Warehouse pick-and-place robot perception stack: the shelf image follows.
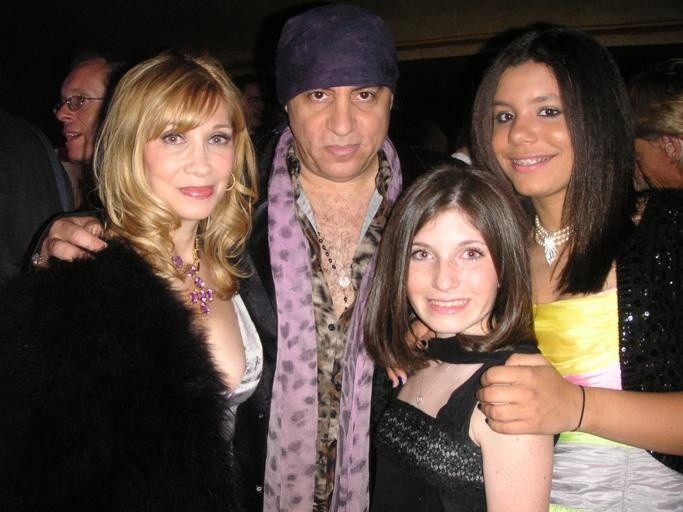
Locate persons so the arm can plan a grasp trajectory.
[1,61,275,284]
[1,50,280,512]
[32,4,474,511]
[471,21,683,511]
[364,165,554,511]
[388,59,682,187]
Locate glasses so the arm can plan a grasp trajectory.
[53,95,111,115]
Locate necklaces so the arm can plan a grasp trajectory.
[170,236,214,321]
